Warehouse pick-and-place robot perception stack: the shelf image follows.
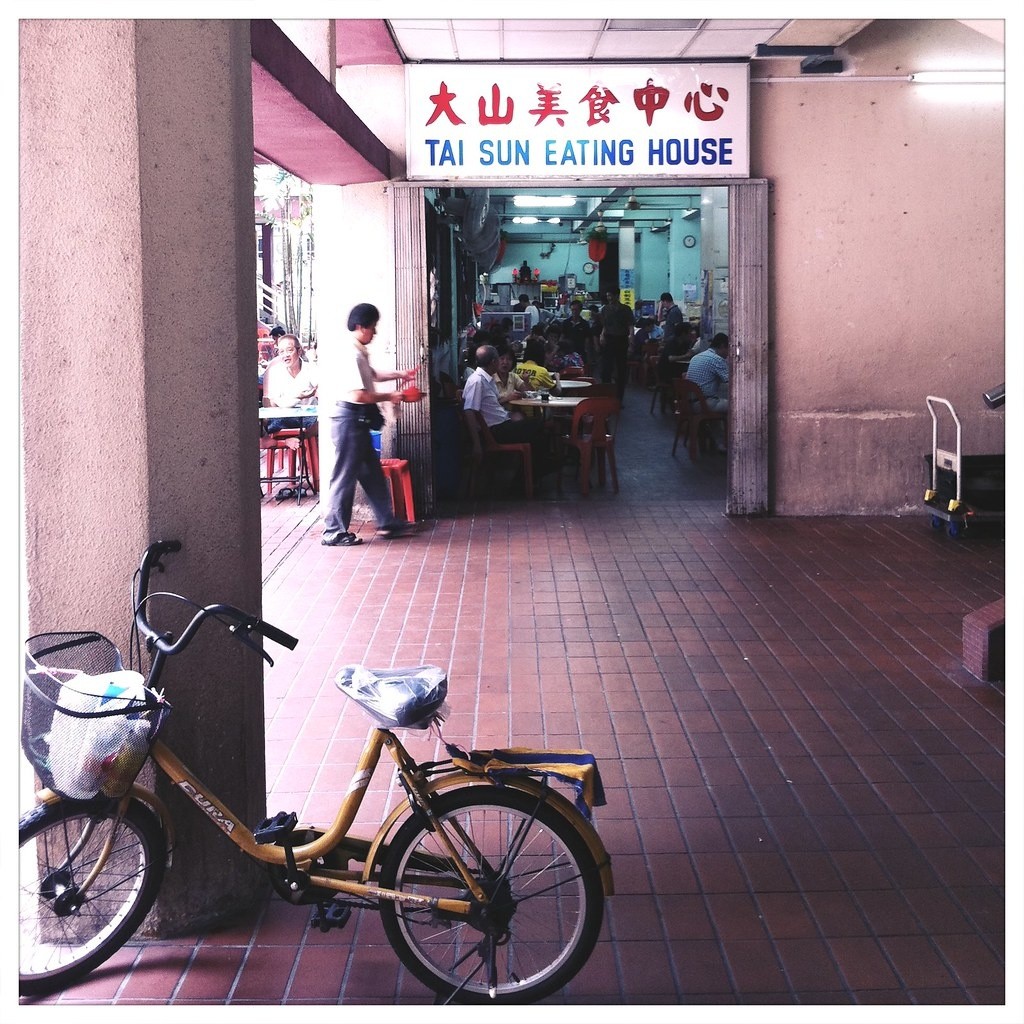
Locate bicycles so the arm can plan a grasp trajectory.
[17,541,616,1005]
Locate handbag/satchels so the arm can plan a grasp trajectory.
[356,404,385,431]
[48,670,146,799]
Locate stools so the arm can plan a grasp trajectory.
[266,427,321,494]
[374,458,416,524]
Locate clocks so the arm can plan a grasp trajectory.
[683,235,696,247]
[584,263,595,274]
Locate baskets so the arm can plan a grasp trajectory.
[20,630,172,804]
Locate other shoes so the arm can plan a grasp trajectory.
[620,404,625,409]
[321,532,363,546]
[376,521,416,539]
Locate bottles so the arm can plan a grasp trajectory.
[101,718,152,798]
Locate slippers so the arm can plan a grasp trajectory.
[293,486,306,498]
[275,487,293,499]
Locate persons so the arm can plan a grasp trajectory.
[685,332,728,453]
[259,326,286,384]
[263,335,316,450]
[321,304,419,546]
[462,287,700,477]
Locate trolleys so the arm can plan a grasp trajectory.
[923,396,1005,537]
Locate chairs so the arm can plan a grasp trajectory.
[458,341,729,499]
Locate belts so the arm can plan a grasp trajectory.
[337,400,362,411]
[693,396,712,403]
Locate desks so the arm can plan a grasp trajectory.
[549,379,593,397]
[507,394,589,473]
[257,405,317,505]
